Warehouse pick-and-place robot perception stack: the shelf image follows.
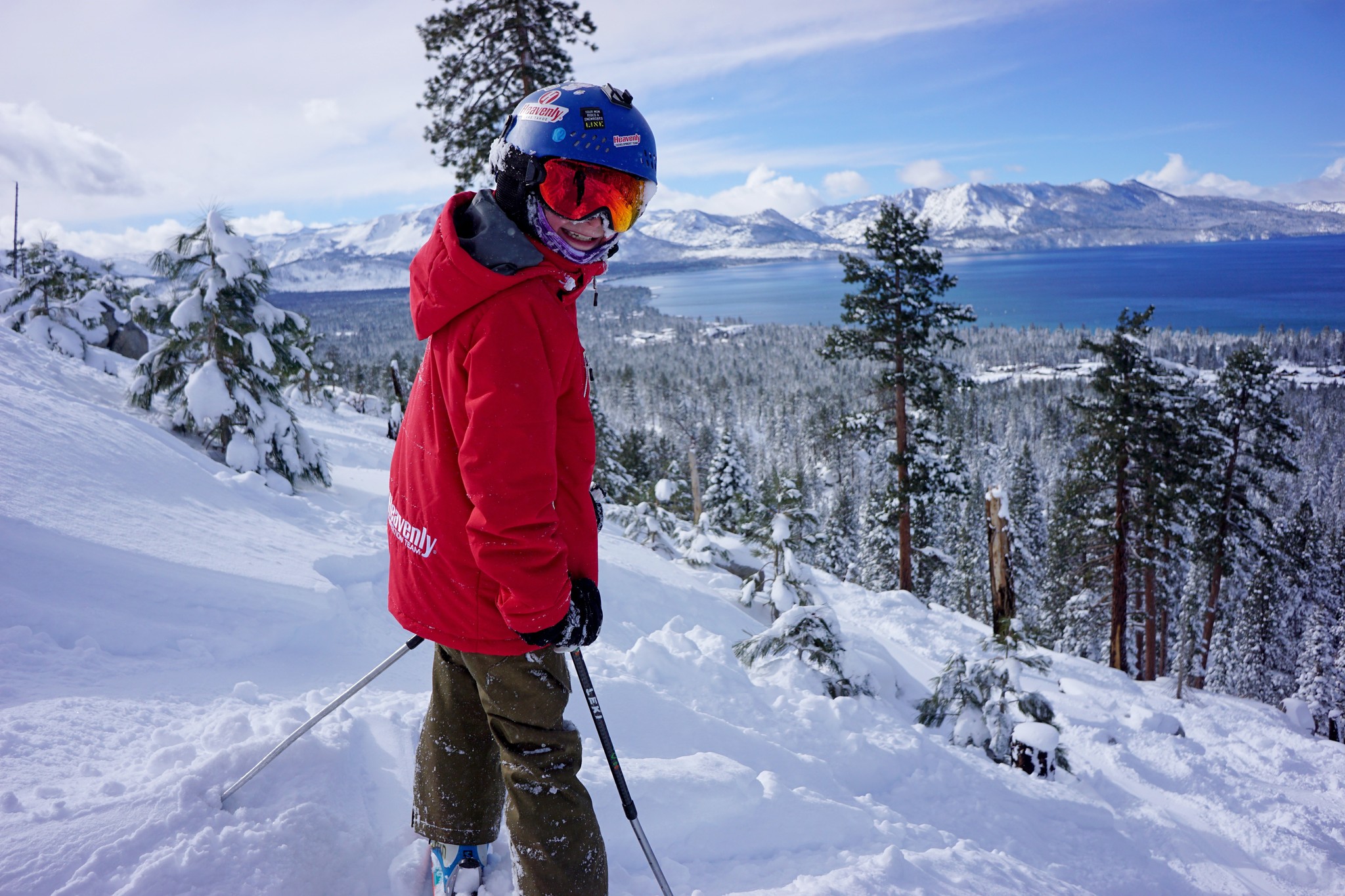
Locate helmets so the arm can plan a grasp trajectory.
[485,80,659,263]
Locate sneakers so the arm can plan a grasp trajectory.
[423,831,496,896]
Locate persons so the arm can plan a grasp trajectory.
[385,78,661,896]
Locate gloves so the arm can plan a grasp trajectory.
[527,573,605,653]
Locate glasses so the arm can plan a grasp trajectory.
[536,159,653,232]
